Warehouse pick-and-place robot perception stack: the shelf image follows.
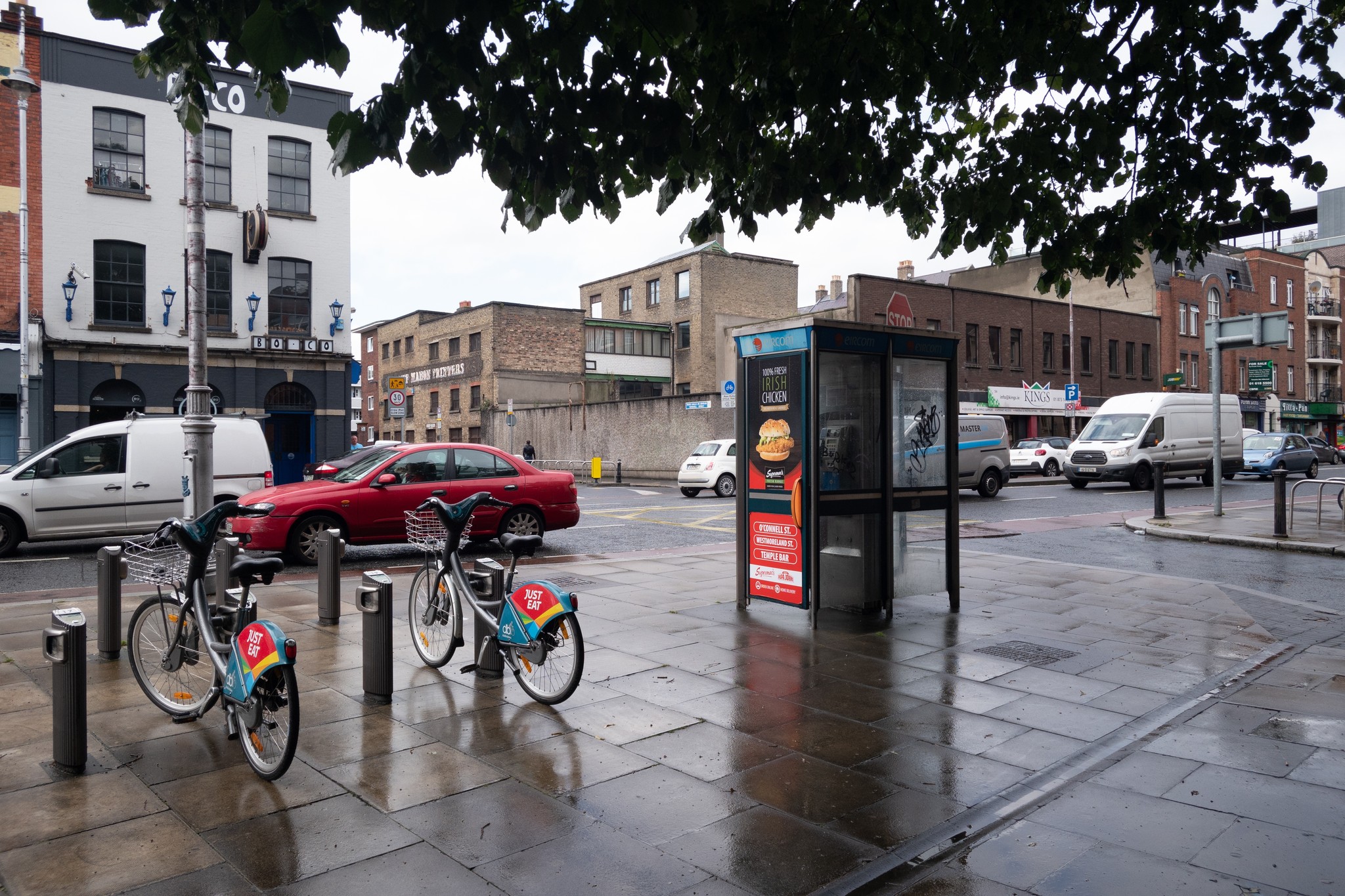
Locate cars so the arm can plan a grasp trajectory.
[1008,436,1073,480]
[1338,441,1345,464]
[224,440,580,569]
[1304,435,1340,465]
[302,439,477,482]
[1242,428,1319,481]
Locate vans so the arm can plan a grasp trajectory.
[1060,392,1243,493]
[677,439,736,498]
[891,413,1012,500]
[0,406,281,561]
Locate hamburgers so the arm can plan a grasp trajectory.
[756,419,794,461]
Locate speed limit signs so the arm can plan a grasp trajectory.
[389,390,405,406]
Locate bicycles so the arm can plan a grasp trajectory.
[403,490,585,706]
[122,496,302,781]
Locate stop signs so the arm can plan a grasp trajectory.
[886,291,913,327]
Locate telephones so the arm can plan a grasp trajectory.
[820,417,862,490]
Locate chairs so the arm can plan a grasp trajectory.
[421,459,437,482]
[1329,348,1338,359]
[1308,303,1316,316]
[1319,389,1333,402]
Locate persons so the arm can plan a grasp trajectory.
[351,436,363,450]
[388,456,426,484]
[1316,431,1327,447]
[83,440,120,474]
[523,440,536,464]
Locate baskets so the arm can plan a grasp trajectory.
[123,529,190,586]
[402,507,476,553]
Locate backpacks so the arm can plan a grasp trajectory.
[525,445,534,455]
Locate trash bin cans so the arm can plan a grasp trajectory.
[591,457,602,479]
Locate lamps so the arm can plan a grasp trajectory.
[161,284,177,327]
[61,279,78,322]
[328,298,344,336]
[245,291,262,332]
